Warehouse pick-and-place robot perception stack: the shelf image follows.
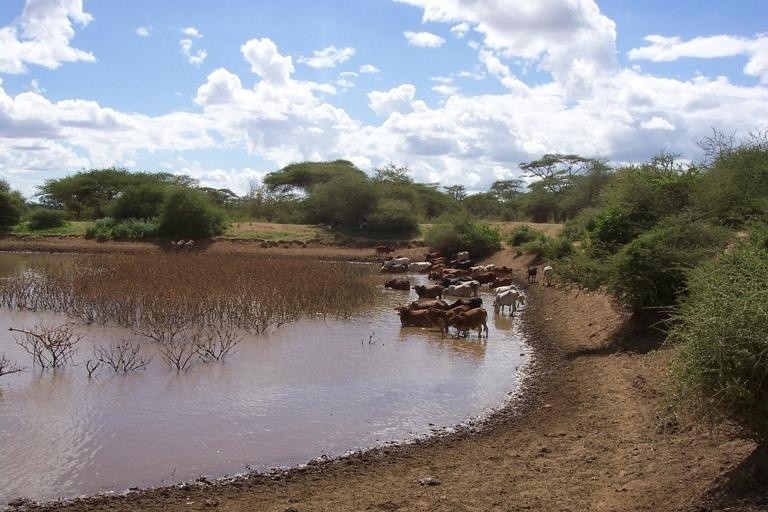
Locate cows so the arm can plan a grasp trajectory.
[543,266,553,287]
[379,251,526,338]
[183,240,195,249]
[375,246,395,257]
[526,267,537,284]
[171,241,177,249]
[177,239,185,248]
